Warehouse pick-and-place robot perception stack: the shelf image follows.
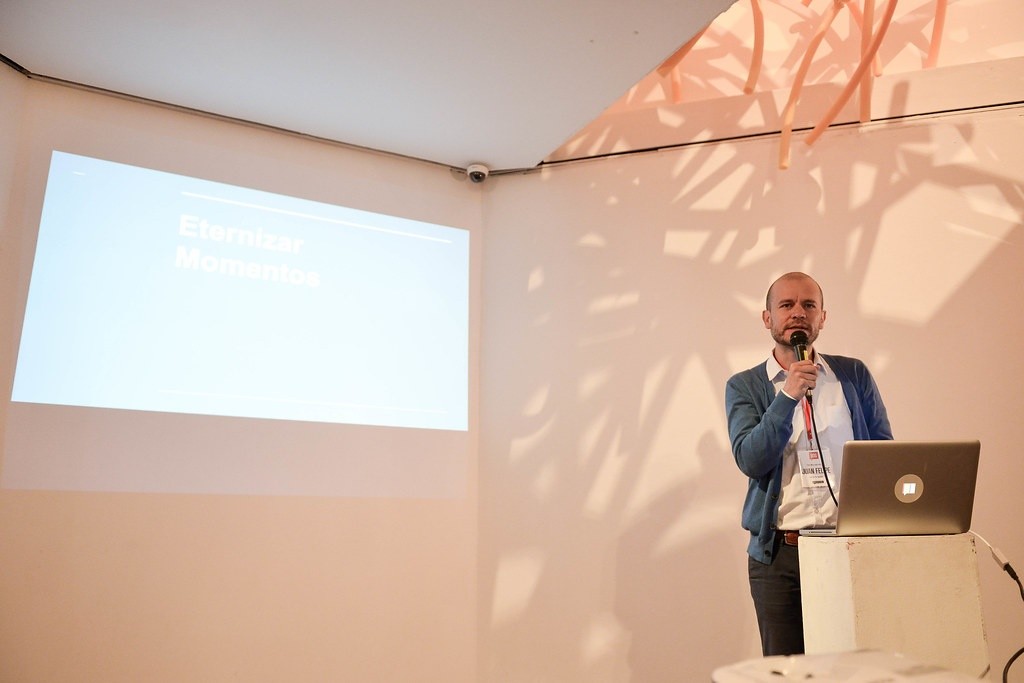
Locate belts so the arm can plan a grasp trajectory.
[773,530,801,546]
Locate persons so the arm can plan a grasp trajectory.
[724,272,894,658]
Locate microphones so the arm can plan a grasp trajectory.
[791,332,811,404]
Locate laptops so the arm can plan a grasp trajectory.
[799,439,982,536]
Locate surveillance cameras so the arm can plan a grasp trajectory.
[467,164,489,182]
